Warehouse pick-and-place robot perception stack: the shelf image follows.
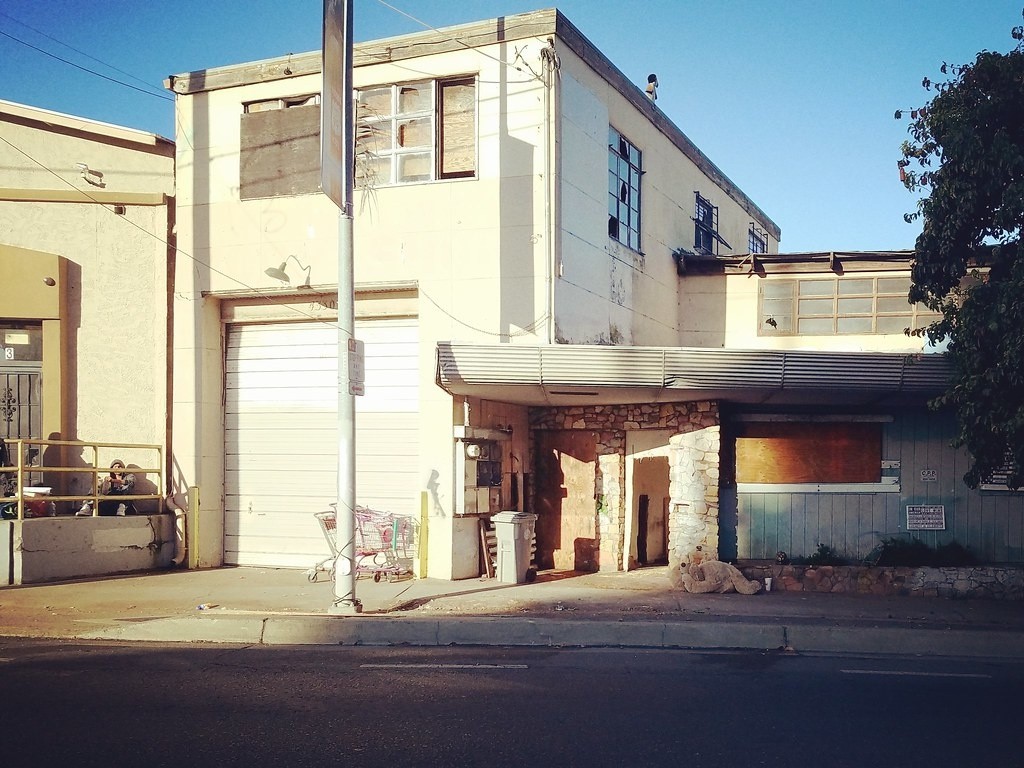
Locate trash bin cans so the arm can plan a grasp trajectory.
[489,511,538,585]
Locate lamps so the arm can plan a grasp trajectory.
[264,255,312,288]
[77,162,106,185]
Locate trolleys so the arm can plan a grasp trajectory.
[306,501,411,583]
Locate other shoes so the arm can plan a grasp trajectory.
[116,503,128,516]
[75,504,92,516]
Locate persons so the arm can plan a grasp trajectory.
[645,74,658,104]
[76,459,136,517]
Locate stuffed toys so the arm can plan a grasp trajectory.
[671,546,762,595]
